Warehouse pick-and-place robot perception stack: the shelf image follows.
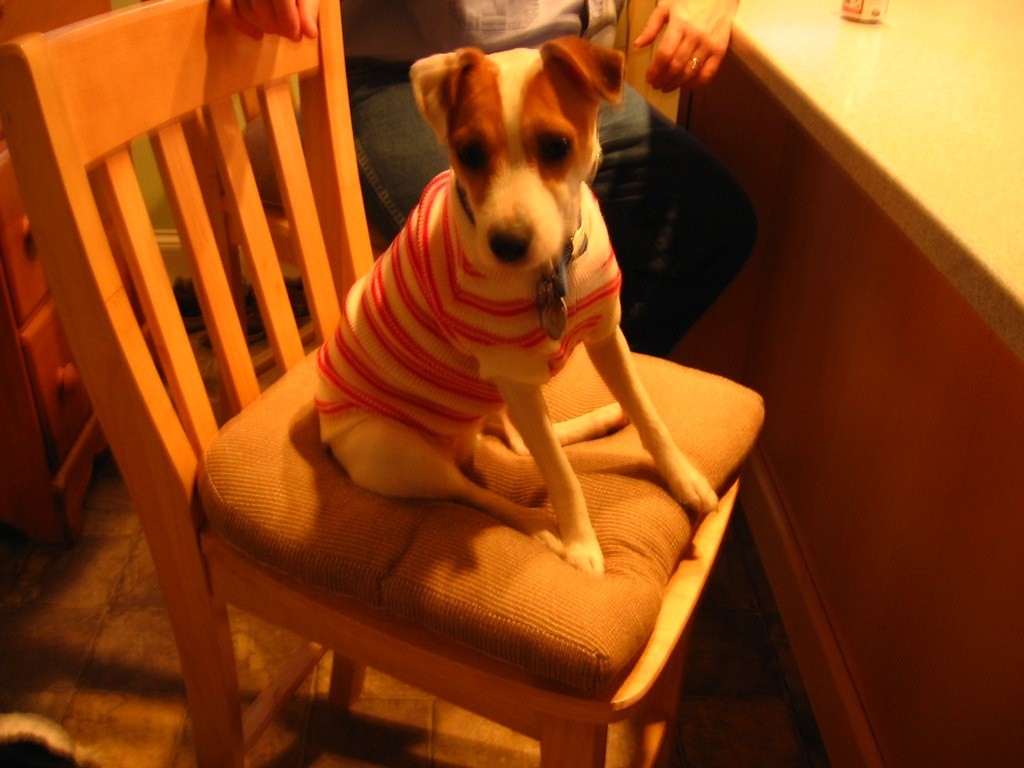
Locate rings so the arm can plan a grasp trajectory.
[690,57,703,70]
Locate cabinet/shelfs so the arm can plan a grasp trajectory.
[0,137,149,544]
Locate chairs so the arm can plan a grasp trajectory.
[0,0,766,768]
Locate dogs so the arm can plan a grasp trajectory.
[313,35,722,576]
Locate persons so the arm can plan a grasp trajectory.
[214,0,760,367]
